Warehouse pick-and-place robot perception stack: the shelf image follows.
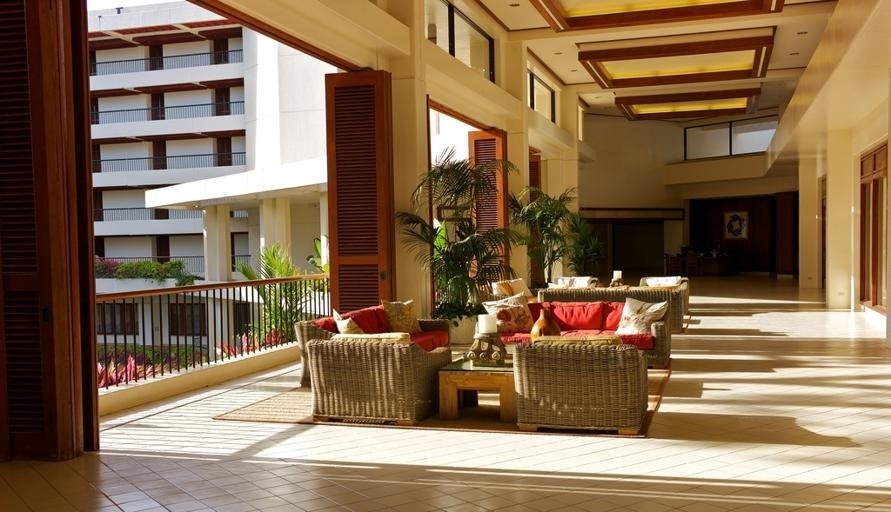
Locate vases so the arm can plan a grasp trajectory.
[530,308,560,342]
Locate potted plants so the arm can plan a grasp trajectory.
[387,146,532,343]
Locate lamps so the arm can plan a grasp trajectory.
[608,270,623,287]
[466,314,507,367]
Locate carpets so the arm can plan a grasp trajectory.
[210,357,672,440]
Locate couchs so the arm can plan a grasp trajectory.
[539,288,684,333]
[500,301,672,368]
[295,303,451,388]
[480,278,536,300]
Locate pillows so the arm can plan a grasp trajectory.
[490,281,512,296]
[332,309,366,334]
[506,279,532,297]
[614,297,669,335]
[479,292,534,332]
[382,299,424,333]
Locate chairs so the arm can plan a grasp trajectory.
[548,277,607,288]
[663,253,699,276]
[518,339,649,435]
[304,339,453,423]
[640,276,690,314]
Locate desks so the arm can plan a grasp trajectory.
[438,352,517,419]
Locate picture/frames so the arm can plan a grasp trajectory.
[723,211,749,239]
